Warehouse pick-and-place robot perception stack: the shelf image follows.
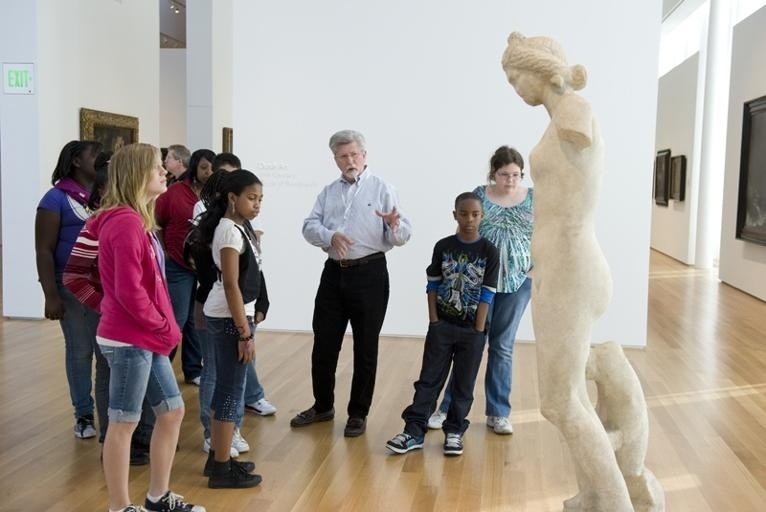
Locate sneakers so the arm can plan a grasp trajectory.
[143,494,207,512]
[427,409,446,428]
[108,503,146,512]
[245,399,276,416]
[183,371,204,385]
[487,414,515,436]
[130,448,147,466]
[386,434,426,451]
[201,436,239,456]
[209,469,260,491]
[229,432,252,449]
[201,459,256,474]
[132,434,178,451]
[75,419,95,439]
[442,428,462,456]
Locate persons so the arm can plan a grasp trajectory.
[385,193,499,456]
[84,144,207,512]
[204,169,263,488]
[154,149,216,386]
[62,150,180,466]
[426,147,533,435]
[166,144,191,180]
[290,131,412,437]
[501,31,666,512]
[183,169,270,458]
[36,141,104,439]
[193,153,278,416]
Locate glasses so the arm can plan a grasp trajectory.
[335,151,365,160]
[496,172,520,178]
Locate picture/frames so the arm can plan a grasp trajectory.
[80,109,139,168]
[656,150,668,206]
[735,94,766,243]
[669,155,687,202]
[223,127,232,156]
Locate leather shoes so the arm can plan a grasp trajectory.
[343,415,368,436]
[291,408,336,427]
